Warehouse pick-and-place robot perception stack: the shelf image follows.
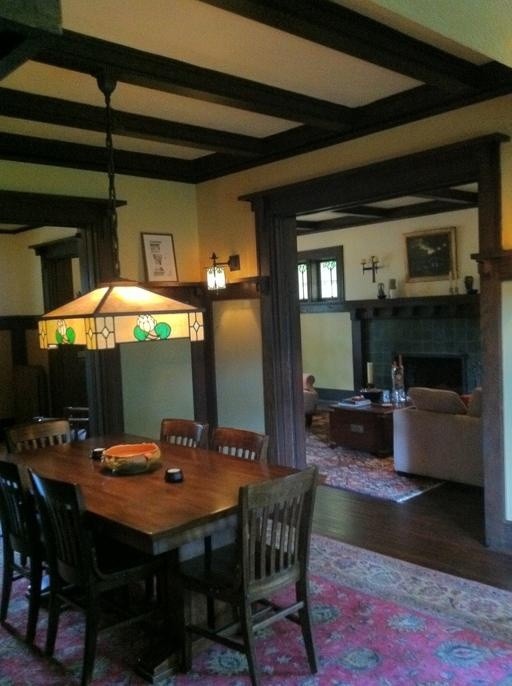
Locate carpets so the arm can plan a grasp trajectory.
[302,411,447,505]
[1,519,511,686]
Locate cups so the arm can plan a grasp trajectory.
[92,448,105,459]
[164,468,183,483]
[383,390,409,403]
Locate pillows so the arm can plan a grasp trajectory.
[467,387,483,418]
[406,387,468,415]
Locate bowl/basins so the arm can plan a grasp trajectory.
[101,442,162,474]
[359,391,383,403]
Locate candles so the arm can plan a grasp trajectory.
[454,269,459,288]
[449,269,453,289]
[366,362,374,384]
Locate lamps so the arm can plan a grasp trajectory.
[360,254,382,282]
[205,252,242,293]
[37,63,209,352]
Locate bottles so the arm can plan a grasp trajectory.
[388,278,397,299]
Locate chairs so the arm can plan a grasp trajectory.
[302,372,319,427]
[164,466,318,686]
[160,418,270,462]
[1,418,171,686]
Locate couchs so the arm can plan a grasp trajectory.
[392,395,484,489]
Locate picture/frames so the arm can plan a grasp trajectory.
[399,226,460,283]
[141,231,180,283]
